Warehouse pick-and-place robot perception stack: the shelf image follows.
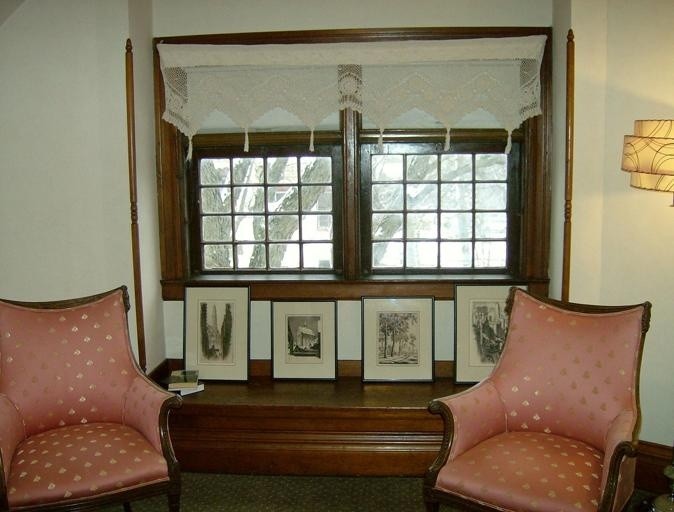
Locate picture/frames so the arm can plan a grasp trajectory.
[455,285,526,383]
[361,296,435,380]
[184,287,251,381]
[271,300,337,380]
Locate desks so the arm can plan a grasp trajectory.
[149,358,478,512]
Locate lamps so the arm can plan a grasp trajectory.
[622,120,674,191]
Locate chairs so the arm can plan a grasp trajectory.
[1,286,181,511]
[422,287,652,511]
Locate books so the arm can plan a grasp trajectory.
[168,370,205,397]
